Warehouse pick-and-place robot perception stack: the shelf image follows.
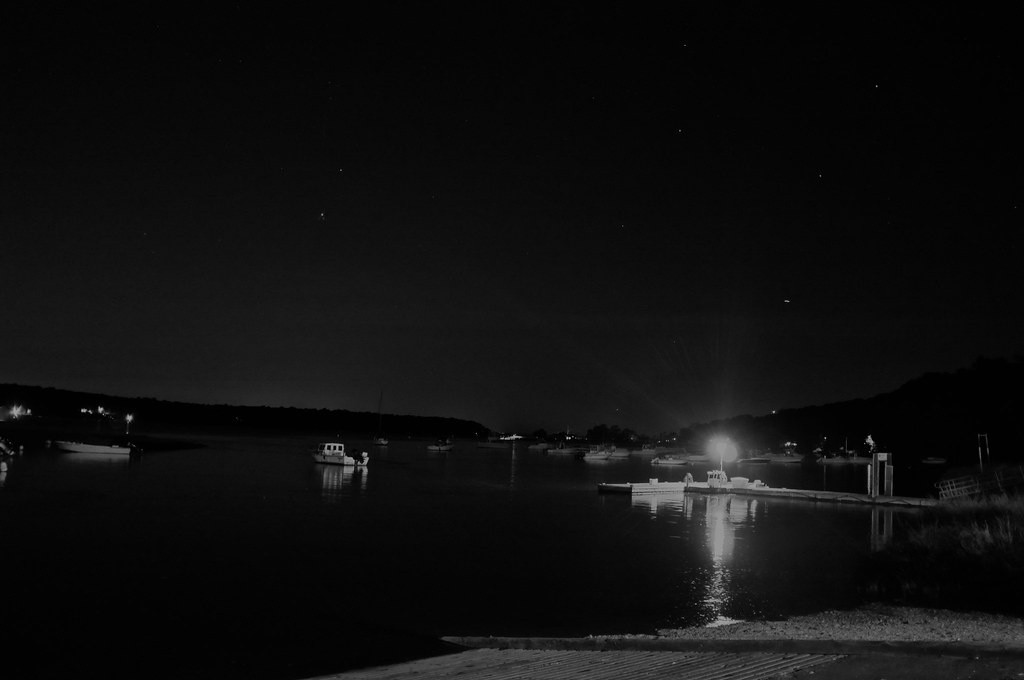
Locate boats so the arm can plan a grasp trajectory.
[584,452,612,461]
[673,453,773,469]
[803,437,872,465]
[651,456,687,469]
[313,442,370,468]
[528,442,553,451]
[371,435,389,446]
[56,439,147,457]
[427,438,455,452]
[476,436,510,449]
[610,449,631,457]
[544,443,590,460]
